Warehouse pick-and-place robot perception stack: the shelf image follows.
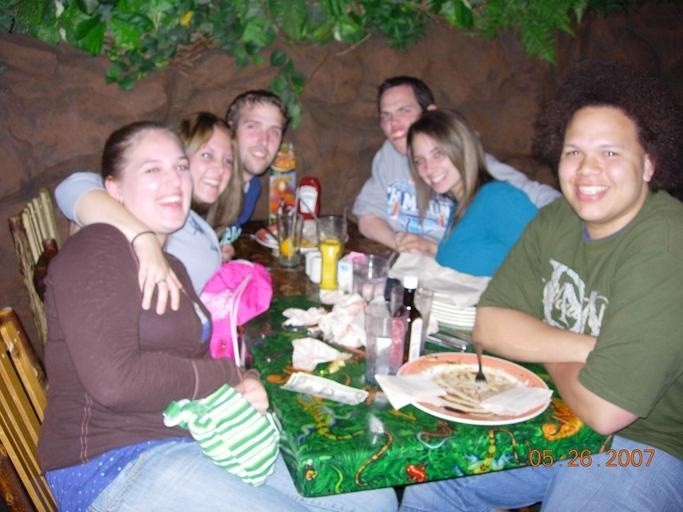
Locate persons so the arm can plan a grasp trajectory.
[390,107,539,309]
[213,88,294,263]
[30,118,399,512]
[50,107,243,316]
[398,59,683,512]
[348,72,566,259]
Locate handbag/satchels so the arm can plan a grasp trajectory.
[161,383,282,487]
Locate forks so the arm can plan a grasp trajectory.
[473,341,490,387]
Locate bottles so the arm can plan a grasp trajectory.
[391,275,423,369]
[268,140,322,221]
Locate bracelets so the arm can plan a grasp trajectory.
[128,231,157,248]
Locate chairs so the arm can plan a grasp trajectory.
[0,306,61,512]
[6,187,62,358]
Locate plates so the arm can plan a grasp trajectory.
[397,351,552,427]
[255,219,348,249]
[411,291,477,334]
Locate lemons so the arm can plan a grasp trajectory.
[280,237,294,257]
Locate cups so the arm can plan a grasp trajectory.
[275,215,412,384]
